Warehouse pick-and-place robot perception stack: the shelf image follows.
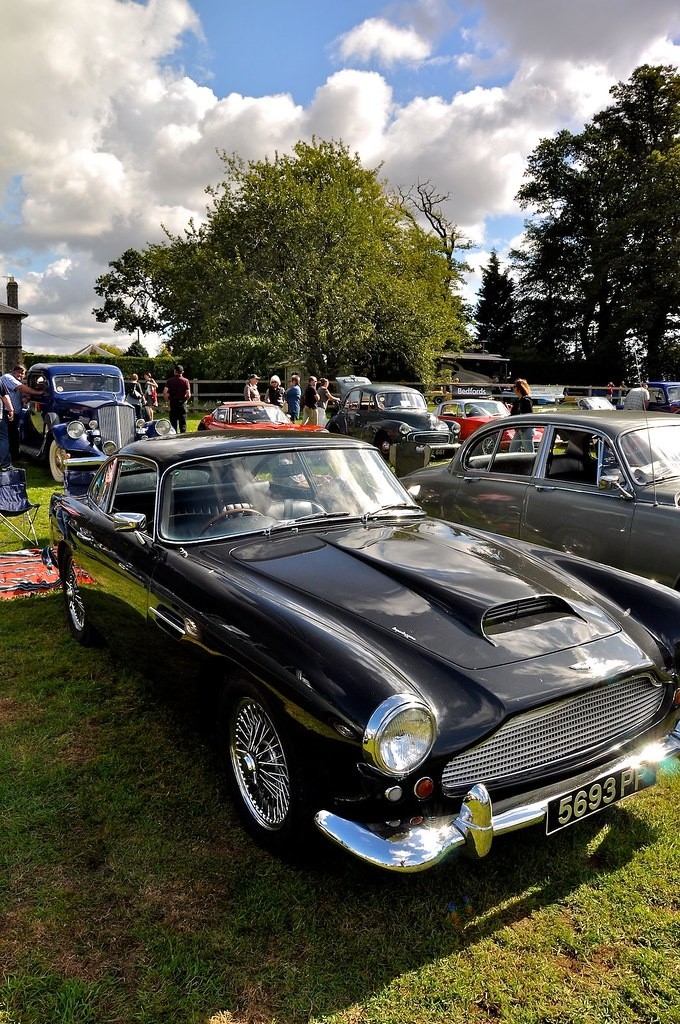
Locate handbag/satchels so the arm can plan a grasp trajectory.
[129,384,142,399]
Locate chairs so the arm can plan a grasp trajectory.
[469,407,486,417]
[382,394,402,409]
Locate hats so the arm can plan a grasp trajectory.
[248,374,260,379]
[270,375,281,386]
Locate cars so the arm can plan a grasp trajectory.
[198,401,329,432]
[18,363,176,484]
[432,398,563,453]
[325,375,462,462]
[398,409,680,592]
[47,429,680,874]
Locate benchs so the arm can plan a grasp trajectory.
[471,454,598,485]
[140,493,327,539]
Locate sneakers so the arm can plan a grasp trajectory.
[1,465,16,471]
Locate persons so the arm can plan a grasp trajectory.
[244,374,261,402]
[163,365,192,434]
[142,372,158,422]
[125,373,146,419]
[264,375,340,427]
[607,382,615,402]
[504,383,512,409]
[623,383,650,411]
[617,381,627,405]
[493,378,502,400]
[0,365,46,472]
[271,451,333,490]
[508,379,534,453]
